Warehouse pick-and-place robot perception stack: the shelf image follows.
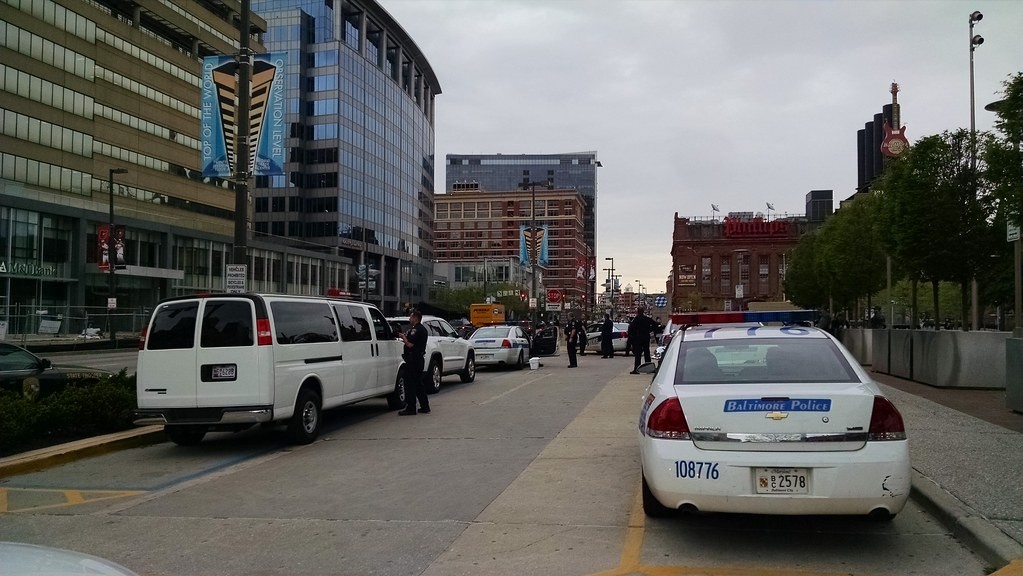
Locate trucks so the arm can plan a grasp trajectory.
[471,304,510,330]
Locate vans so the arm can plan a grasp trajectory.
[133,291,408,444]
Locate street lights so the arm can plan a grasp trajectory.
[606,257,615,321]
[967,9,986,331]
[109,167,130,344]
[636,280,647,308]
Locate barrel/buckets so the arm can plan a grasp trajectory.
[529,356,540,370]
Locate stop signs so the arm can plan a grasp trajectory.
[548,290,562,303]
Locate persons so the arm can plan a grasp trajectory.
[398,311,431,416]
[818,306,850,329]
[577,264,585,280]
[99,240,109,264]
[115,238,124,260]
[871,306,886,330]
[625,307,665,374]
[536,315,559,329]
[564,312,588,368]
[601,313,614,359]
[589,265,596,280]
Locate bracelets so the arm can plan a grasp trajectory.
[570,337,573,338]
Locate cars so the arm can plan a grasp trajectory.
[463,324,560,370]
[575,321,630,354]
[0,337,117,393]
[656,318,687,346]
[636,310,912,526]
[0,539,140,576]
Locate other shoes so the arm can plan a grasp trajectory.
[580,353,586,356]
[398,408,417,416]
[568,365,577,368]
[630,370,640,374]
[601,356,608,359]
[609,355,613,358]
[623,353,629,357]
[417,407,430,413]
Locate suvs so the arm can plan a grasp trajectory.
[374,316,476,394]
[449,319,476,340]
